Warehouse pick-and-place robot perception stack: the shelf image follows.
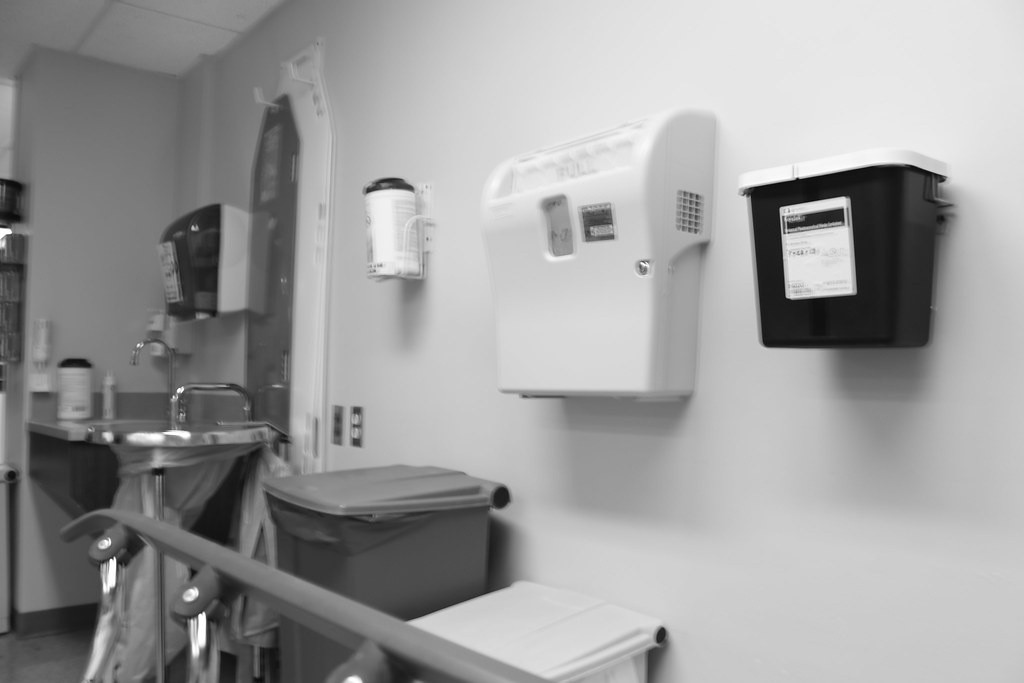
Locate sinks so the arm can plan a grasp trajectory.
[84,419,272,449]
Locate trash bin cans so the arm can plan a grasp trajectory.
[260,462,512,683]
[405,581,666,683]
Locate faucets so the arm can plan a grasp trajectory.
[130,337,174,419]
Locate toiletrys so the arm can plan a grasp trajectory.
[101,367,116,420]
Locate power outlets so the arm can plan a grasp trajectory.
[333,406,341,445]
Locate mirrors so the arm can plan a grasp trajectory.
[239,37,333,473]
